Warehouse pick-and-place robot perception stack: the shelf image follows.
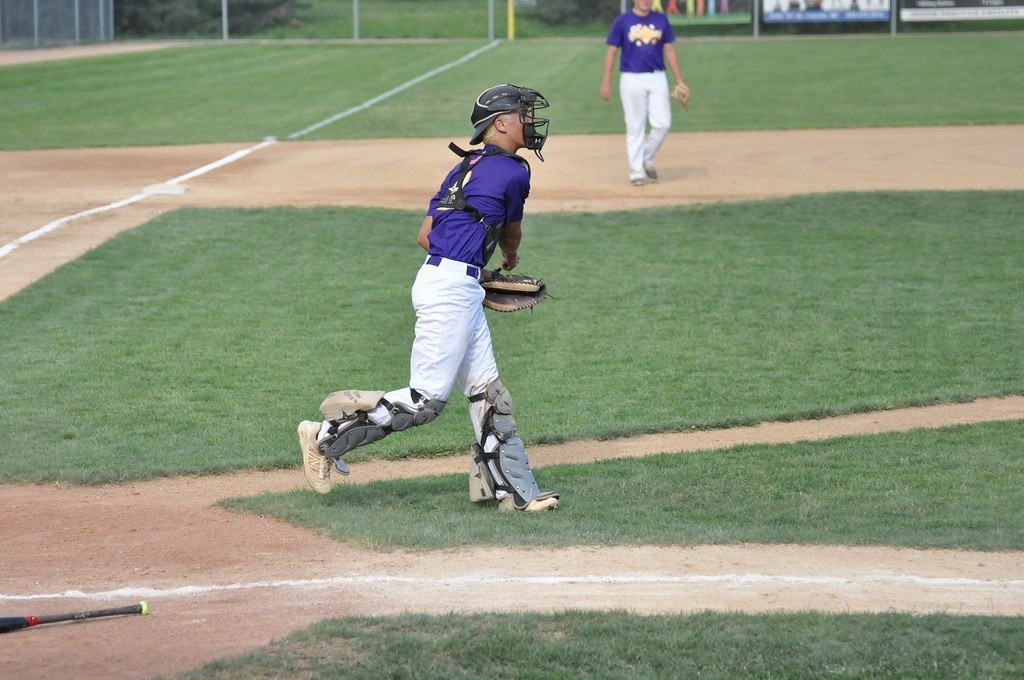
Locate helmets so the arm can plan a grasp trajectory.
[469,84,522,145]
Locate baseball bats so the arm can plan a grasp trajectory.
[0,601,149,634]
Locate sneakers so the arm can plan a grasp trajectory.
[498,495,559,514]
[298,420,332,495]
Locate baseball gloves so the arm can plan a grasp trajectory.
[670,77,691,108]
[478,272,547,314]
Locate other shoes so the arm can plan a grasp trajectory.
[643,161,658,179]
[633,179,643,187]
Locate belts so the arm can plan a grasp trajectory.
[427,257,479,279]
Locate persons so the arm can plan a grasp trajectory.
[297,84,561,512]
[599,0,689,186]
